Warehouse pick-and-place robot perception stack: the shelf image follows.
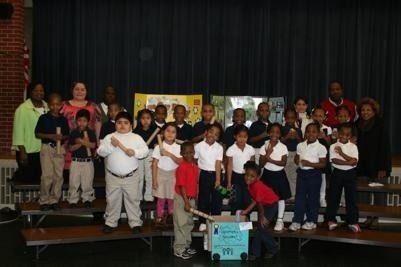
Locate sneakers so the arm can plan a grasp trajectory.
[348,224,361,233]
[71,200,91,209]
[199,224,207,232]
[103,224,114,234]
[40,203,59,212]
[327,222,337,230]
[174,248,197,259]
[133,226,141,234]
[250,244,279,260]
[274,220,284,231]
[154,215,172,224]
[302,222,317,230]
[289,222,301,230]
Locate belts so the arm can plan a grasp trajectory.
[107,169,137,178]
[44,141,64,147]
[72,157,91,162]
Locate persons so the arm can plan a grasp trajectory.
[9,77,393,235]
[238,160,281,262]
[172,141,200,261]
